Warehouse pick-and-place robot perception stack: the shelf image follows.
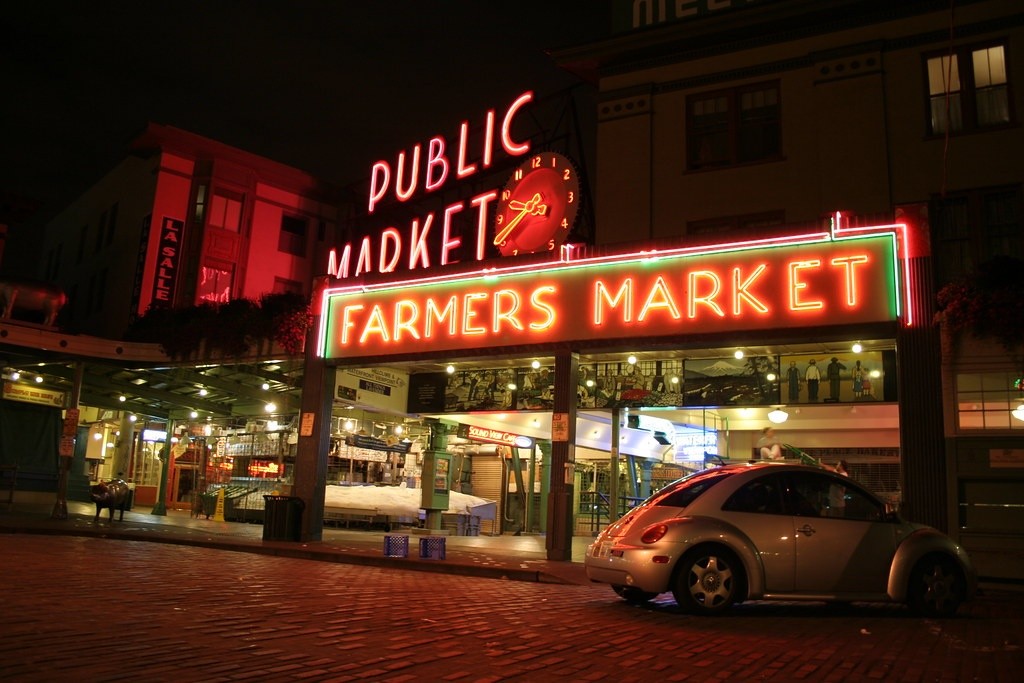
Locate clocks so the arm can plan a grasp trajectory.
[495,150,578,257]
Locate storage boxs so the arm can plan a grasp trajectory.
[384,536,408,558]
[418,535,445,560]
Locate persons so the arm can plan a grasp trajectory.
[819,459,848,517]
[758,427,781,460]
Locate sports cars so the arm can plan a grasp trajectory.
[584,459,973,620]
